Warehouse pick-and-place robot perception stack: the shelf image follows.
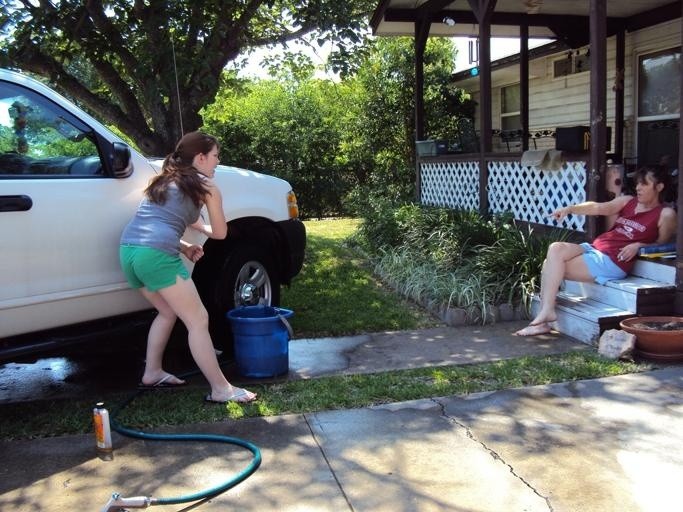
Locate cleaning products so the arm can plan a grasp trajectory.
[92,401,112,452]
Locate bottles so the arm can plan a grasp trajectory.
[93,402,111,450]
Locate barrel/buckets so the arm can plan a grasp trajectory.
[227,304,294,380]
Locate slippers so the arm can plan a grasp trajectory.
[139,371,186,390]
[203,388,260,404]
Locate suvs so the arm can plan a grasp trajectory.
[0,68,306,364]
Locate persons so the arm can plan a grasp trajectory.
[517,168,675,337]
[118,132,259,404]
